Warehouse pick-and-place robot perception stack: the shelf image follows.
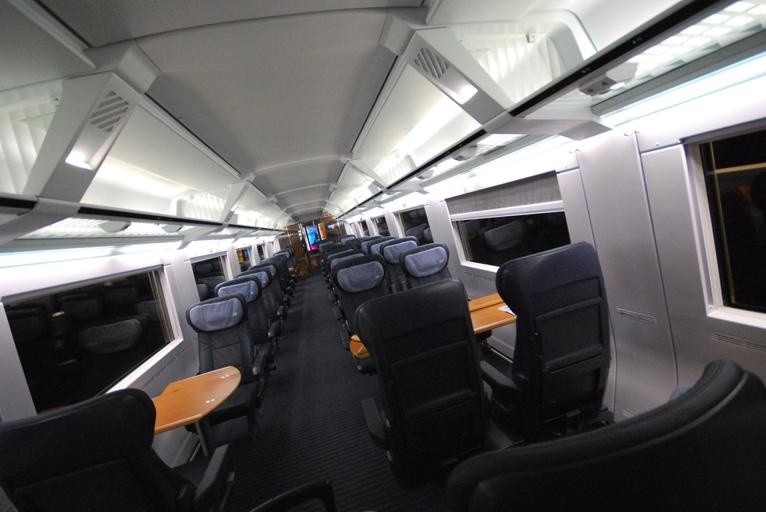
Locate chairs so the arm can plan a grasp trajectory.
[0,233,766,512]
